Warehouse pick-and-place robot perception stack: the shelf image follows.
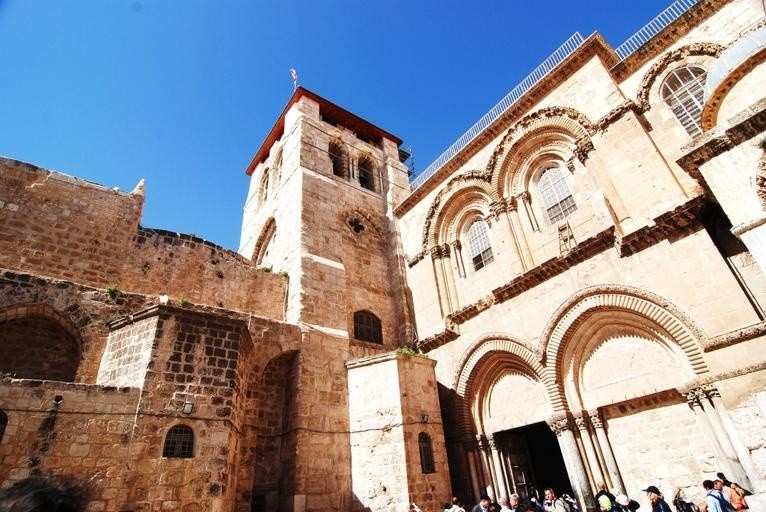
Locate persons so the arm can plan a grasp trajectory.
[409,473,748,512]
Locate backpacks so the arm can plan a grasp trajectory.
[709,492,738,512]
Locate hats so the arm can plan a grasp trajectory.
[642,486,660,495]
